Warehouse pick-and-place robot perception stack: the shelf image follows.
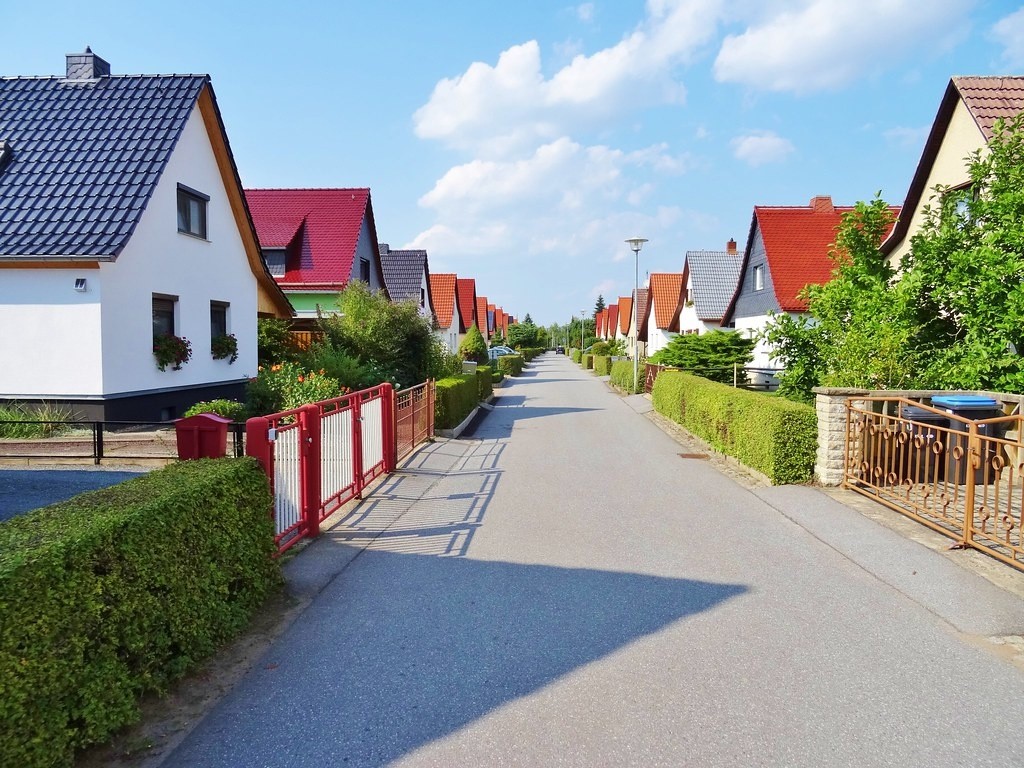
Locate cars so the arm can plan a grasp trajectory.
[488,346,521,356]
[556,346,565,355]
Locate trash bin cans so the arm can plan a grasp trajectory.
[894,406,945,483]
[930,396,1003,485]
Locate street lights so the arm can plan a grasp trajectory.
[566,323,570,348]
[580,308,587,354]
[623,234,649,394]
